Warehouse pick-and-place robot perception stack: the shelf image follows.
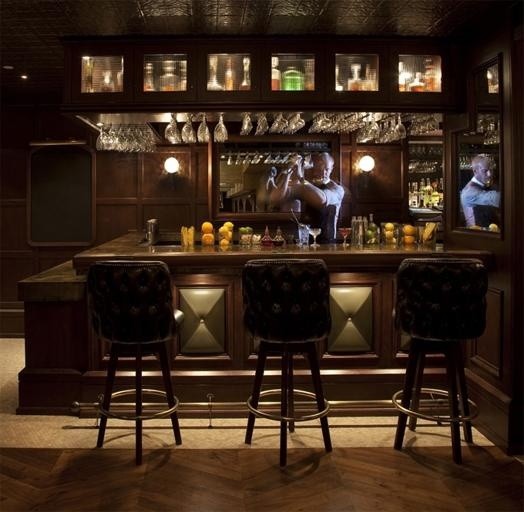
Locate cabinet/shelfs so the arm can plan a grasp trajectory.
[59,31,464,145]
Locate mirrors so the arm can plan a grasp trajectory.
[213,133,343,223]
[448,51,504,240]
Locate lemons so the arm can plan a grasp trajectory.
[225,231,232,240]
[385,223,394,231]
[219,226,229,235]
[224,221,234,230]
[385,231,394,240]
[221,239,229,245]
[386,238,396,244]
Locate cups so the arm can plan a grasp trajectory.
[201,228,215,247]
[146,219,161,245]
[400,226,418,252]
[218,230,233,247]
[299,225,309,246]
[93,122,157,153]
[365,227,380,244]
[308,228,322,247]
[241,112,306,136]
[411,114,440,138]
[382,228,399,252]
[423,229,438,248]
[239,230,249,247]
[309,113,407,143]
[339,228,351,247]
[164,113,228,143]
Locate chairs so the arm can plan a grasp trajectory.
[241,258,333,467]
[392,258,488,464]
[87,260,185,466]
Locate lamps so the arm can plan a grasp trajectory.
[164,157,179,175]
[359,155,374,175]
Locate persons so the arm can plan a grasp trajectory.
[269,152,346,244]
[258,155,301,212]
[461,153,500,228]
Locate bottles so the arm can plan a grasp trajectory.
[399,62,410,92]
[261,226,272,246]
[336,64,343,91]
[356,216,363,244]
[272,57,281,90]
[239,58,250,90]
[348,64,364,91]
[351,216,356,243]
[117,59,123,91]
[272,227,285,247]
[282,65,304,90]
[304,59,314,90]
[408,177,443,208]
[365,64,377,90]
[369,214,373,226]
[207,56,223,90]
[409,71,427,92]
[159,60,177,90]
[363,216,368,243]
[225,57,234,90]
[177,61,186,90]
[82,57,94,92]
[99,70,115,92]
[423,59,436,91]
[144,63,156,91]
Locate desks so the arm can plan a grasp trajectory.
[16,258,85,414]
[73,230,492,416]
